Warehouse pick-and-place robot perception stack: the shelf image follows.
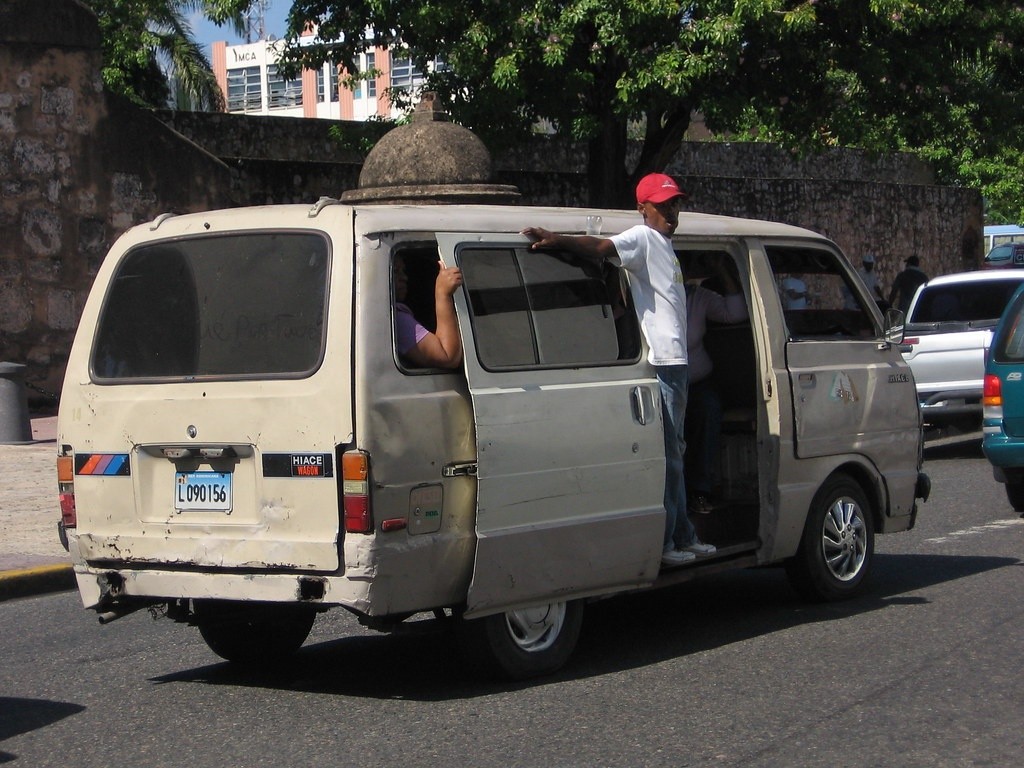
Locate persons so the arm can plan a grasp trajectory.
[675,250,750,513]
[862,260,886,310]
[839,273,860,310]
[888,255,929,315]
[524,173,716,565]
[783,273,812,308]
[392,255,463,369]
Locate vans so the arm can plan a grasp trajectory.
[57,198,938,685]
[900,266,1024,431]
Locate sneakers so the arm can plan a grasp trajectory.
[661,549,694,565]
[677,542,717,557]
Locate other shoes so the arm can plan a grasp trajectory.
[690,493,713,513]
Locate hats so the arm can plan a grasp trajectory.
[863,255,874,263]
[636,172,689,204]
[903,256,919,266]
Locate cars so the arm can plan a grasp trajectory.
[982,223,1024,270]
[979,281,1024,516]
